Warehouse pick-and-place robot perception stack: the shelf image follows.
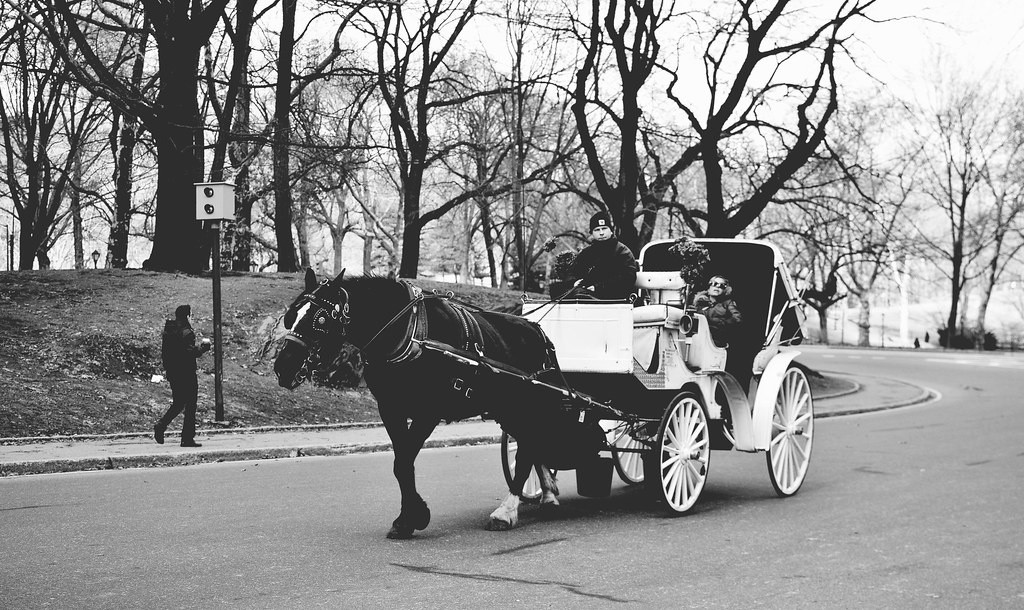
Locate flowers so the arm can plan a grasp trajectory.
[667,236,712,287]
[550,252,578,283]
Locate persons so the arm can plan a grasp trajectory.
[550,211,637,305]
[693,276,743,347]
[152,304,212,448]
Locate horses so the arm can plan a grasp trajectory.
[272,265,563,542]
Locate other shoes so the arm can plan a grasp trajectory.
[180,439,202,447]
[154,424,164,444]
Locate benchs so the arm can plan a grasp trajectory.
[686,311,785,376]
[631,270,690,364]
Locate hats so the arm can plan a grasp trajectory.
[589,211,613,235]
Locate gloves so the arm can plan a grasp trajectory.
[715,306,727,316]
[200,342,211,351]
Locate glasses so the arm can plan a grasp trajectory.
[708,282,726,290]
[188,314,194,317]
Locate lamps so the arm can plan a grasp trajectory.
[92,250,100,269]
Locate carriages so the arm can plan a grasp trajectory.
[268,233,815,540]
